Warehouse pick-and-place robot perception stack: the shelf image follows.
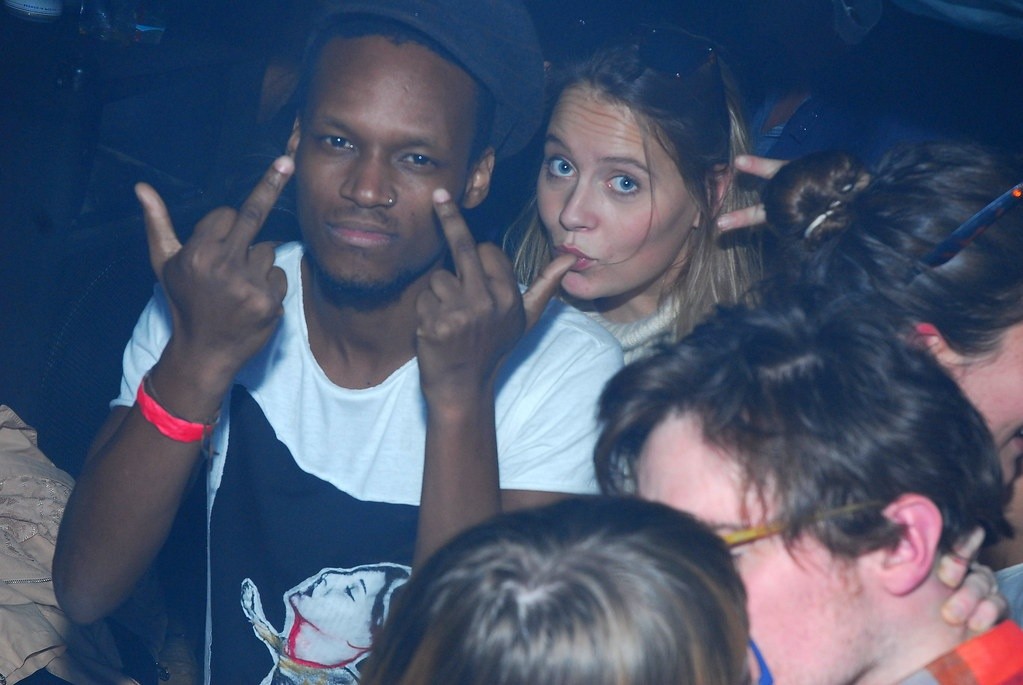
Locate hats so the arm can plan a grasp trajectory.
[302,0,547,163]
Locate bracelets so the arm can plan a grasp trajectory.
[137,374,220,443]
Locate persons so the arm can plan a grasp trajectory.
[591,266,1023,685]
[353,494,1010,685]
[501,20,793,366]
[763,138,1023,629]
[51,0,626,685]
